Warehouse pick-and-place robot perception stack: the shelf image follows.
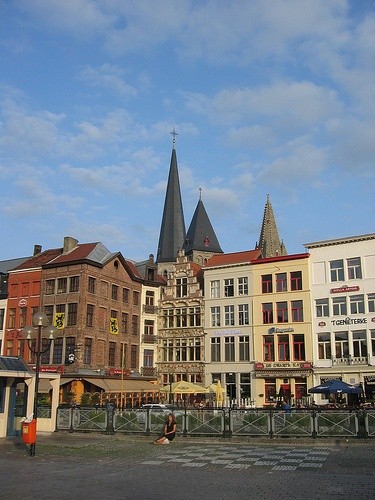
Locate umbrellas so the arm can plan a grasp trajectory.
[308,380,362,408]
[159,381,210,393]
[206,384,226,393]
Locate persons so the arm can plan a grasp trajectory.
[118,399,375,413]
[152,413,177,445]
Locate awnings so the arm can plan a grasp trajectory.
[16,378,74,393]
[82,378,159,393]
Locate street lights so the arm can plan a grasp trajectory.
[22,311,59,457]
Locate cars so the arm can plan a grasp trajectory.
[135,404,190,424]
